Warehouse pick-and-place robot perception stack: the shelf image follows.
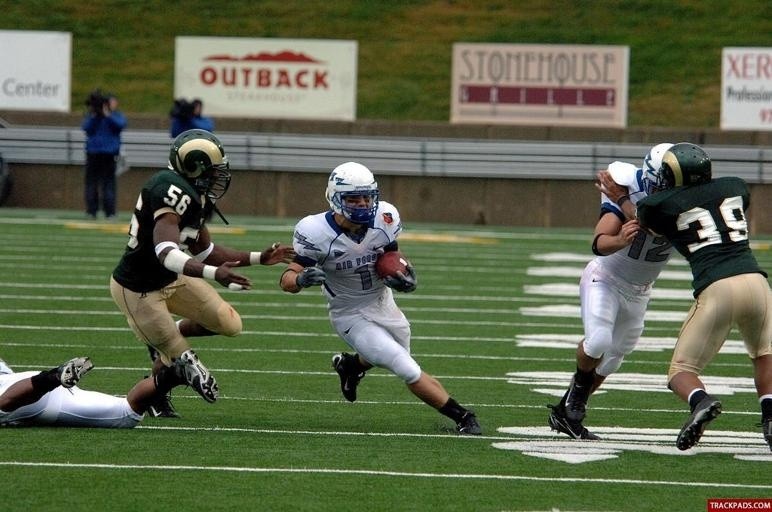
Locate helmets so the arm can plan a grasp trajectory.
[324,161,378,223]
[641,143,712,196]
[169,129,231,199]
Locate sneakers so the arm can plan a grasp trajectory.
[331,352,364,402]
[178,350,219,403]
[55,357,93,388]
[565,374,589,421]
[756,419,771,451]
[546,405,600,440]
[456,411,482,435]
[148,395,182,418]
[677,396,721,451]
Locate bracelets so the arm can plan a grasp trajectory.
[248,251,263,266]
[202,264,220,281]
[615,195,630,206]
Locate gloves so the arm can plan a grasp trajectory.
[384,264,418,293]
[296,267,327,288]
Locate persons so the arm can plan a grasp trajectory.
[278,160,486,437]
[1,346,223,431]
[170,98,216,139]
[108,127,299,419]
[80,93,131,222]
[545,141,678,442]
[594,139,770,454]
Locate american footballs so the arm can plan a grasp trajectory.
[376,251,409,278]
